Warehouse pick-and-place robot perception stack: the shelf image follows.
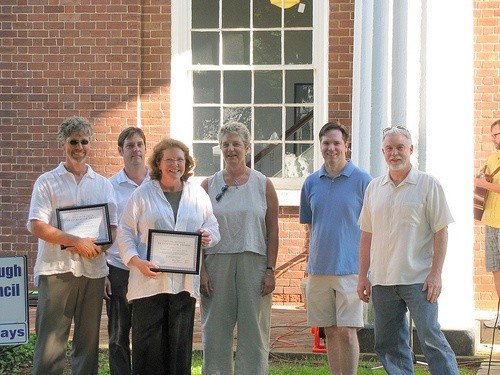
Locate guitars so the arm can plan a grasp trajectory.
[473,170,494,221]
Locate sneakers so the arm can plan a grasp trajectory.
[484,319,500,330]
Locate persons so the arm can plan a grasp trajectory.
[117,137,220,375]
[27,117,117,375]
[103,126,155,375]
[297,123,374,375]
[472,119,500,328]
[358,126,462,374]
[197,121,277,375]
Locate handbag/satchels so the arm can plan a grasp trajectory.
[474,165,500,221]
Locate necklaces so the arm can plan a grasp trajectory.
[226,170,247,186]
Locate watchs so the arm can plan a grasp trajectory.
[266,266,276,271]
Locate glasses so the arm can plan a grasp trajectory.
[65,139,90,145]
[162,158,184,162]
[383,126,410,137]
[216,185,229,201]
[491,133,500,138]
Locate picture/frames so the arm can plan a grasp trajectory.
[293,83,314,157]
